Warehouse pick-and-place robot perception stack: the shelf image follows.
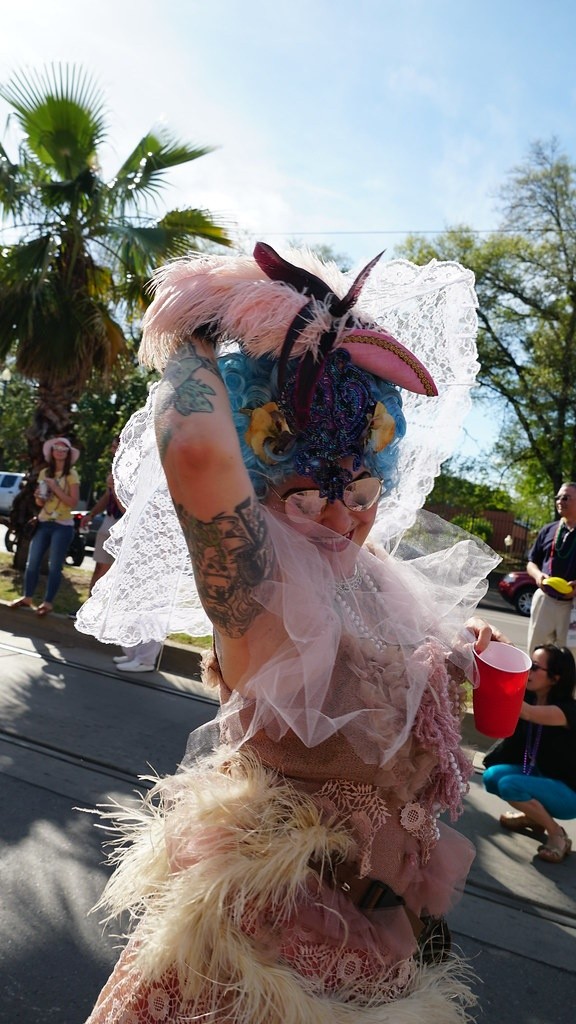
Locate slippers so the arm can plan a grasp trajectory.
[34,606,54,616]
[8,599,30,607]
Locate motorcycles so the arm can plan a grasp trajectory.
[5,513,86,566]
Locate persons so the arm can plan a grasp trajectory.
[524,481,575,652]
[483,644,576,862]
[73,247,572,1024]
[10,437,126,613]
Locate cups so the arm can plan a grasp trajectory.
[472,641,532,738]
[35,480,48,501]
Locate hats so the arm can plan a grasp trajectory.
[72,240,482,647]
[42,438,80,465]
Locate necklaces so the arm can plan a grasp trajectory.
[43,476,67,515]
[329,559,403,654]
[522,724,543,777]
[548,517,564,575]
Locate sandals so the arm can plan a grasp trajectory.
[537,826,572,861]
[500,811,543,832]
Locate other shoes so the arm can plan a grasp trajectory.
[68,611,77,619]
[117,659,155,673]
[113,655,129,663]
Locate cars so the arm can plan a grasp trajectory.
[500,572,538,618]
[71,510,107,548]
[0,471,29,515]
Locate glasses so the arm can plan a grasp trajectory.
[531,663,550,673]
[52,445,69,452]
[554,495,574,502]
[268,457,384,524]
